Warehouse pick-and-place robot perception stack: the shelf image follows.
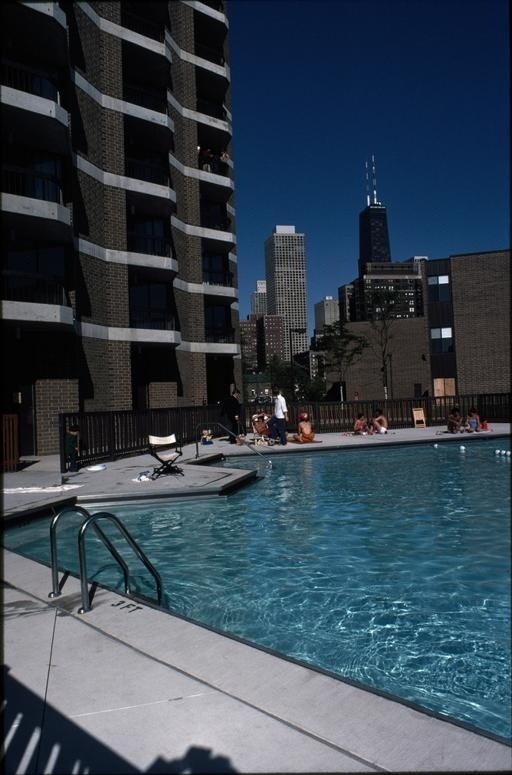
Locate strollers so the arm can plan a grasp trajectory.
[252,414,288,445]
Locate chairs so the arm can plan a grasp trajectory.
[144,433,187,481]
[248,414,285,447]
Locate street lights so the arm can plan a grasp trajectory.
[289,328,306,374]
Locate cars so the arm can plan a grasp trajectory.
[413,387,429,406]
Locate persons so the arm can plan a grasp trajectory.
[266,386,289,445]
[200,429,214,445]
[227,388,241,443]
[64,425,80,472]
[351,391,359,400]
[252,408,489,445]
